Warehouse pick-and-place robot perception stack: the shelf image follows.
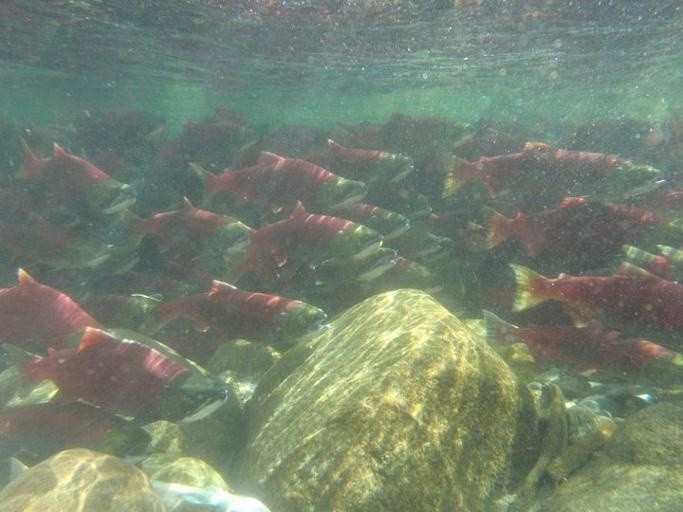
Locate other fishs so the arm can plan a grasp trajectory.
[0,403,151,457]
[0,110,682,377]
[0,326,228,430]
